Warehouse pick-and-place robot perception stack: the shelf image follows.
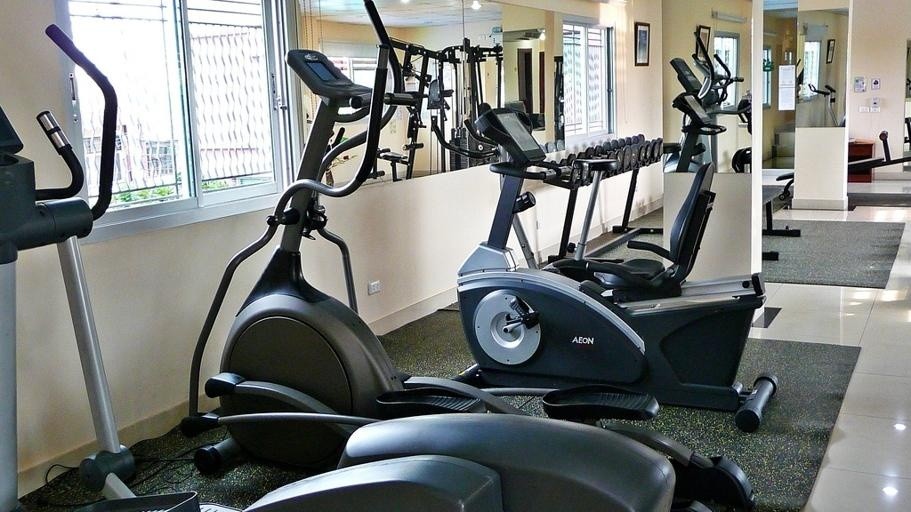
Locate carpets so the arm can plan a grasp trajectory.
[761,183,909,219]
[586,211,909,291]
[71,303,865,505]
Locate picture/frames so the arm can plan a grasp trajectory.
[694,23,712,67]
[635,21,650,67]
[825,39,836,63]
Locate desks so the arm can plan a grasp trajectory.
[848,139,876,183]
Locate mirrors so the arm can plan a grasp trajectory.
[662,0,753,172]
[795,6,849,129]
